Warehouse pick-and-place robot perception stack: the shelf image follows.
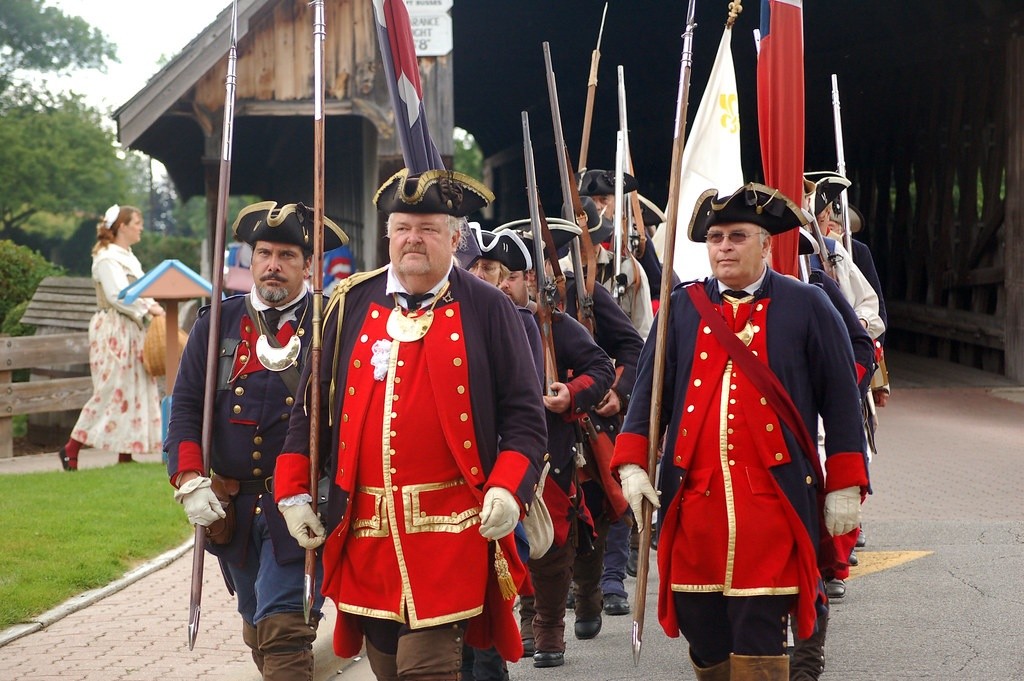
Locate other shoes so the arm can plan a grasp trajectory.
[59,446,79,472]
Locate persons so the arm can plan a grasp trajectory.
[163,202,349,681]
[466,170,683,681]
[797,171,891,681]
[610,184,865,680]
[58,206,161,472]
[275,168,547,681]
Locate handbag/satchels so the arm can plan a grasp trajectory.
[142,315,189,378]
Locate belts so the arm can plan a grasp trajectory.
[235,478,279,495]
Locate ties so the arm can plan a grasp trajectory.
[723,292,755,320]
[259,296,304,337]
[397,291,434,312]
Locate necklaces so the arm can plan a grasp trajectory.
[255,293,309,372]
[386,281,450,342]
[723,293,755,347]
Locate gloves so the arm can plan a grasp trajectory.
[475,490,521,543]
[278,495,325,551]
[176,475,225,527]
[821,484,864,538]
[618,462,662,532]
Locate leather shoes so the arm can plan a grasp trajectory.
[510,514,867,681]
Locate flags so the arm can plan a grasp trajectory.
[758,0,804,279]
[372,0,445,172]
[651,25,744,283]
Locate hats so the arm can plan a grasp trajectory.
[801,177,817,196]
[467,221,532,272]
[561,196,614,246]
[626,193,667,227]
[233,200,349,253]
[492,217,582,261]
[830,198,865,233]
[372,166,496,217]
[575,169,641,195]
[803,171,852,215]
[686,181,809,243]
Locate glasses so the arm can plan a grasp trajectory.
[705,230,766,245]
[467,263,500,275]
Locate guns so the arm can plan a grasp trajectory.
[753,28,853,285]
[520,41,640,409]
